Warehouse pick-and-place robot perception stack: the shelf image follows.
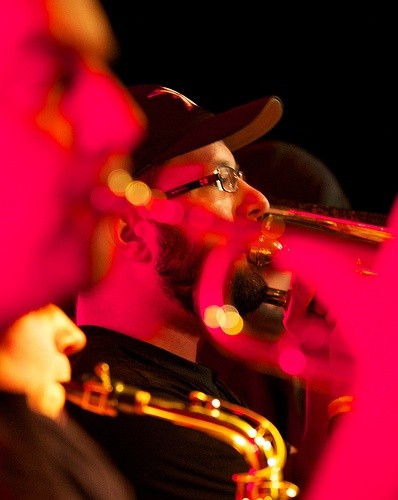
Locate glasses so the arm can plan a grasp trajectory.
[155,165,246,199]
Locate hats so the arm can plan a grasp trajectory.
[123,85,283,178]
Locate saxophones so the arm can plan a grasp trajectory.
[249,207,395,430]
[64,360,302,500]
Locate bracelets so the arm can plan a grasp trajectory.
[328,395,353,417]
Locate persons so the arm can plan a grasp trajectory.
[61,88,353,500]
[0,0,398,500]
[231,142,348,309]
[0,304,87,418]
